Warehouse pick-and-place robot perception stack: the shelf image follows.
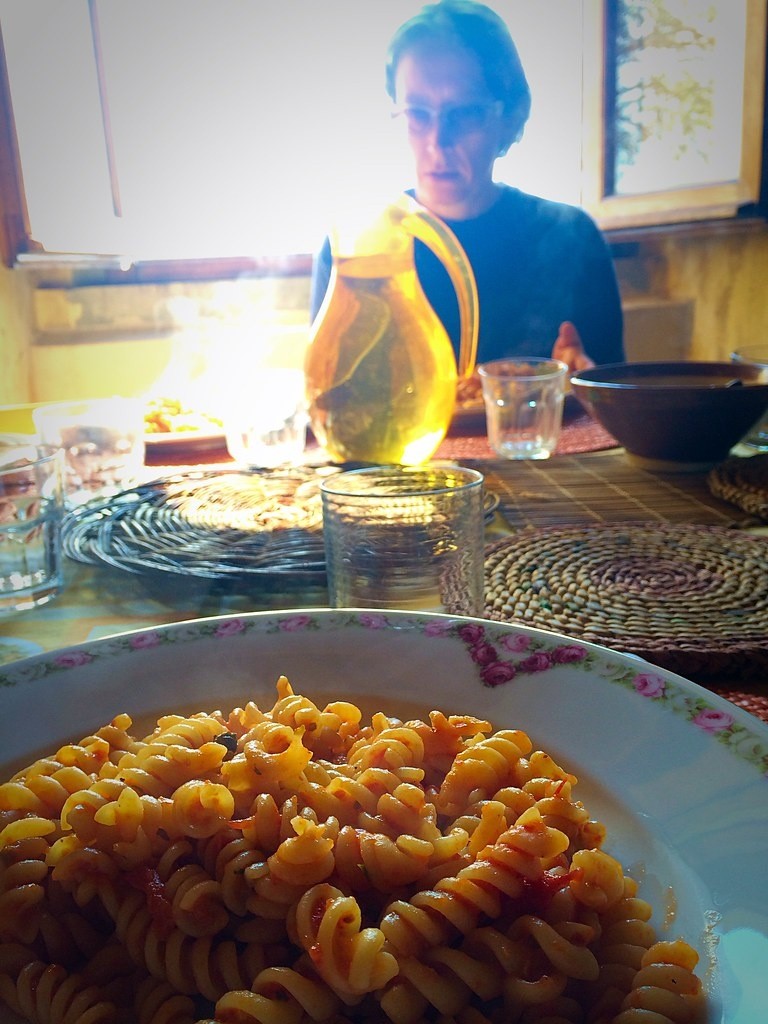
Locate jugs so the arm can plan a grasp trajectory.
[302,185,480,467]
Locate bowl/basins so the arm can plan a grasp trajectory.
[570,360,768,474]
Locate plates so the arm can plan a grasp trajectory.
[0,608,768,1024]
[146,434,227,454]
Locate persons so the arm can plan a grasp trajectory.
[309,1,627,428]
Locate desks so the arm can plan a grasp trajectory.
[0,432,768,721]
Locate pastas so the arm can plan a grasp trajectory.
[0,675,707,1024]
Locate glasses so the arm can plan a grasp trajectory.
[390,98,505,133]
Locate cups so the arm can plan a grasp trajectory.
[320,464,484,621]
[34,399,145,513]
[218,364,306,468]
[477,357,569,460]
[0,438,64,614]
[731,345,768,452]
